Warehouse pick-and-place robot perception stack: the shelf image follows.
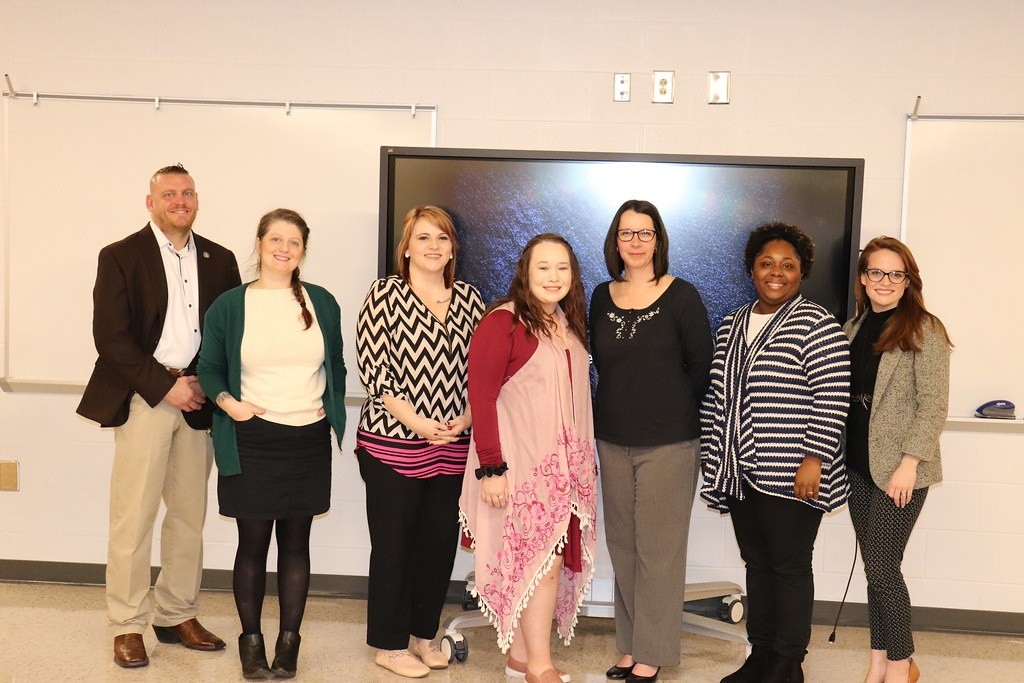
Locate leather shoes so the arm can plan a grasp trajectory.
[151,617,226,650]
[114,633,149,667]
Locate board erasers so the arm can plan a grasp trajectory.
[974,400,1017,419]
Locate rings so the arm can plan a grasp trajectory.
[808,492,814,494]
[499,496,503,499]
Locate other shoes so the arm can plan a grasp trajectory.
[375,648,430,678]
[504,655,571,683]
[626,663,660,683]
[523,665,564,683]
[605,662,636,679]
[412,637,448,669]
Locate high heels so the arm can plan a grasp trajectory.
[907,657,920,683]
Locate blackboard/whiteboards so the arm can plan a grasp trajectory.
[1,92,437,408]
[898,114,1023,426]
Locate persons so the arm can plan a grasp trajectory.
[456,232,602,683]
[77,162,242,669]
[843,238,953,683]
[587,199,714,683]
[698,224,854,683]
[353,206,487,681]
[194,211,348,683]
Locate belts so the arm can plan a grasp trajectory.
[166,367,187,377]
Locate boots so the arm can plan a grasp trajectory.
[238,631,270,678]
[718,648,805,683]
[271,630,302,677]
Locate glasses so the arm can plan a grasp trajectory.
[617,229,657,242]
[864,267,908,284]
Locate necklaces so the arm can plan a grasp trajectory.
[436,292,452,305]
